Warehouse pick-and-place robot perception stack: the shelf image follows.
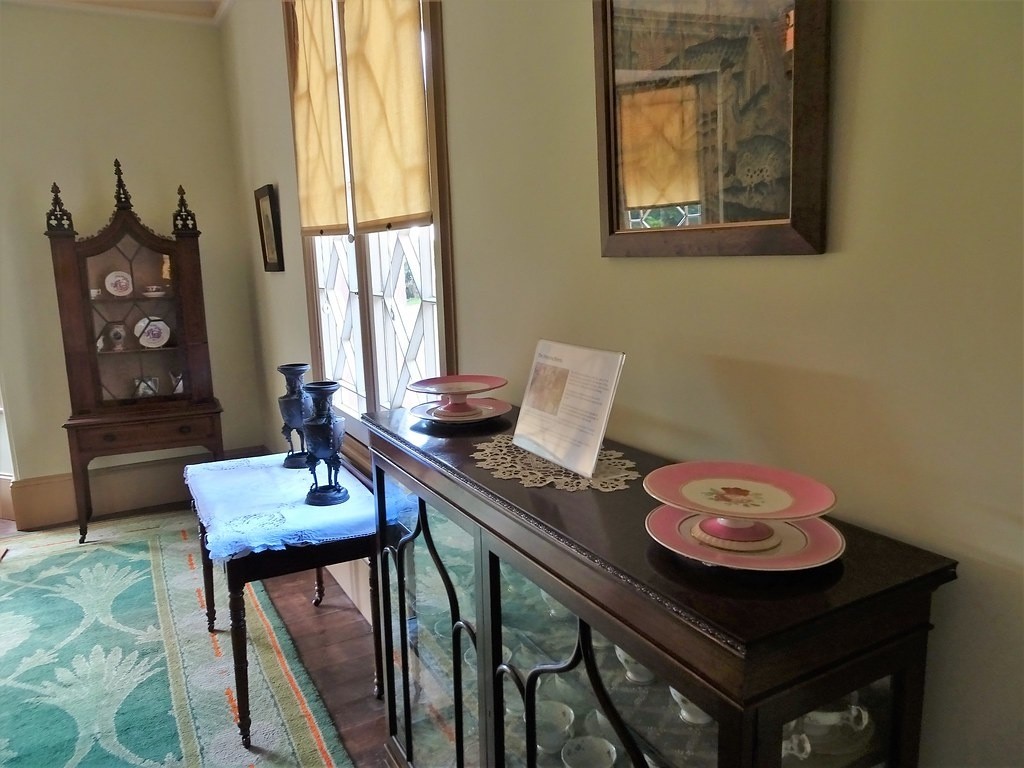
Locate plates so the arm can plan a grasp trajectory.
[134,316,171,348]
[105,271,134,297]
[143,291,167,298]
[103,321,132,349]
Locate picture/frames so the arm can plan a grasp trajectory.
[587,0,838,262]
[253,184,286,274]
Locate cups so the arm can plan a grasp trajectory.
[90,288,101,299]
[143,285,162,292]
[402,543,867,767]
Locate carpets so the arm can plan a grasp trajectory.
[0,521,358,767]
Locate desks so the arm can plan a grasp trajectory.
[183,446,413,746]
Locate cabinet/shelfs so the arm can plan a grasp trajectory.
[41,159,229,539]
[351,390,963,767]
